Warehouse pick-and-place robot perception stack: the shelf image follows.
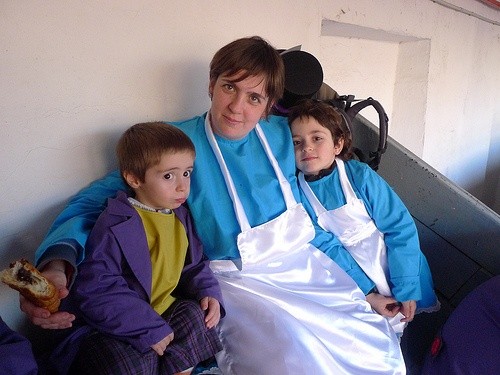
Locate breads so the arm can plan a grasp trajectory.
[0,259,60,314]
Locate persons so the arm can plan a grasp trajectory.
[17,35,407,375]
[286,102,443,375]
[72,121,227,374]
[422,276,500,375]
